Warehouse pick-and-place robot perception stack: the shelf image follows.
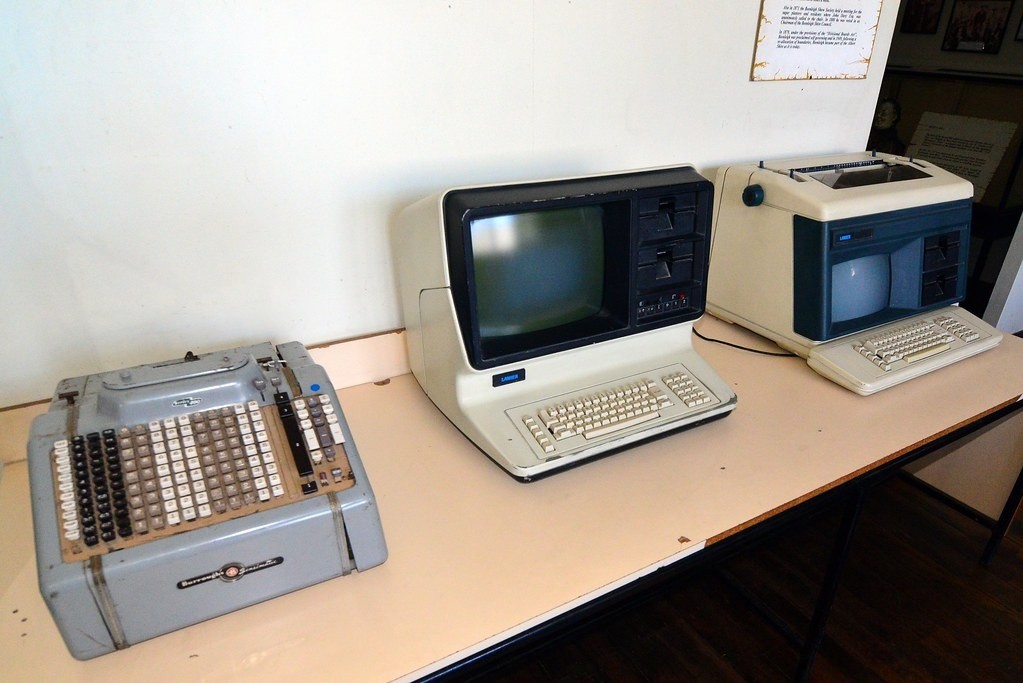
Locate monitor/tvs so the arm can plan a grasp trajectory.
[831,253,890,324]
[468,205,606,340]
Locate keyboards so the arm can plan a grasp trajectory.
[503,363,723,460]
[854,315,979,371]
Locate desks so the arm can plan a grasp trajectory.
[0,315,1023,683]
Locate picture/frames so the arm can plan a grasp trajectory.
[900,0,945,35]
[941,0,1015,55]
